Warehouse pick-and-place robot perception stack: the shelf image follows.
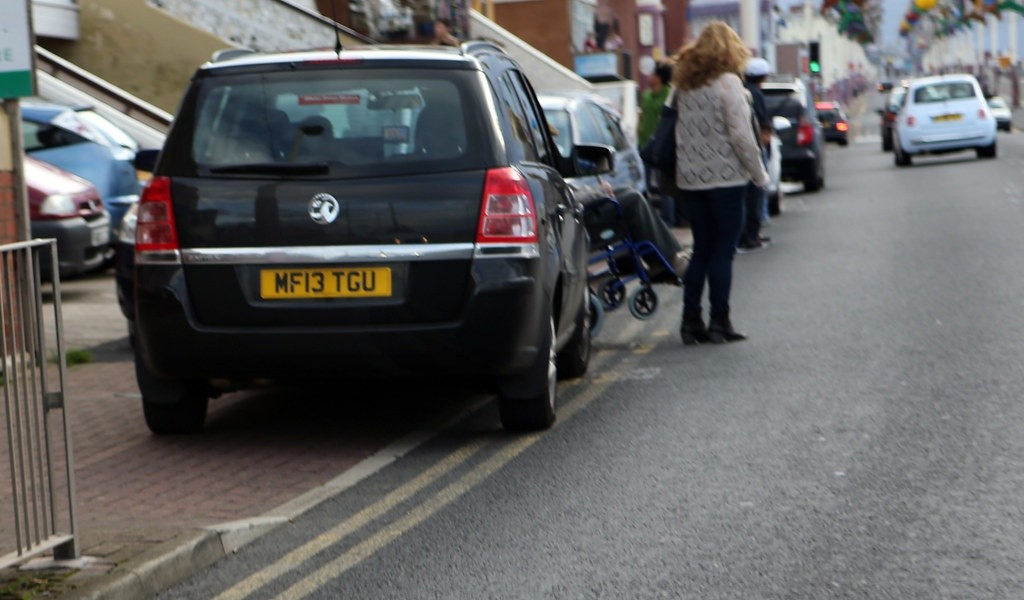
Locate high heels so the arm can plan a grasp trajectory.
[708,306,747,343]
[681,307,709,345]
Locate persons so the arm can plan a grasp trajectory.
[548,124,694,278]
[735,56,770,252]
[676,20,771,346]
[639,47,693,227]
[415,5,440,44]
[637,58,674,151]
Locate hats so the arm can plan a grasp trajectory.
[745,57,770,75]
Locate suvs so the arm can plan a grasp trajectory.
[130,39,594,435]
[739,79,825,194]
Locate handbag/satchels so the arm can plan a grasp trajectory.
[641,86,677,165]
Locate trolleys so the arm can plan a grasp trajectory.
[578,200,689,318]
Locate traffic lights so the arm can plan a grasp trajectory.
[807,40,822,80]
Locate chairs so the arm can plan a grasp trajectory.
[410,101,461,155]
[227,109,341,163]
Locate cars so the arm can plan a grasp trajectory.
[536,93,648,219]
[874,75,1015,168]
[814,100,850,152]
[13,94,159,300]
